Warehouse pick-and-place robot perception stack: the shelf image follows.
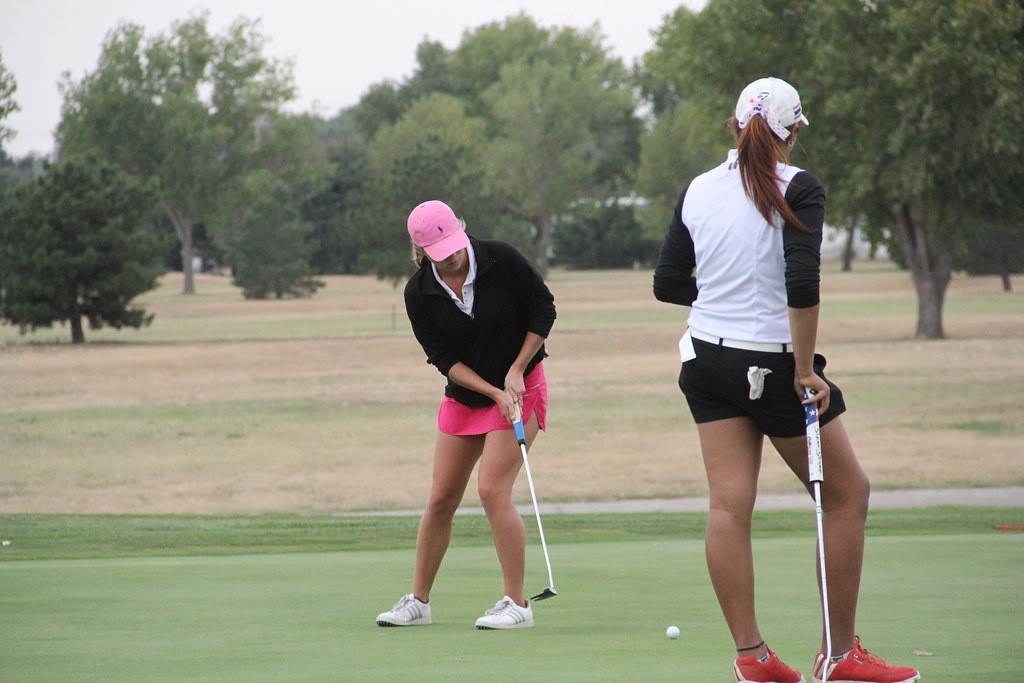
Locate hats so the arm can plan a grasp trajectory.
[736,77,810,141]
[407,200,470,262]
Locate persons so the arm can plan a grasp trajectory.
[375,199,557,630]
[653,76,921,683]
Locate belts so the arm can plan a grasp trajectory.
[679,326,793,363]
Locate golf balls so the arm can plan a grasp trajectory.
[666,625,680,639]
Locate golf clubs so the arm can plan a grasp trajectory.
[507,389,558,602]
[803,386,832,683]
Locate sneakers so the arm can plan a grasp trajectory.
[475,595,535,630]
[812,636,921,683]
[376,594,432,625]
[733,644,807,683]
